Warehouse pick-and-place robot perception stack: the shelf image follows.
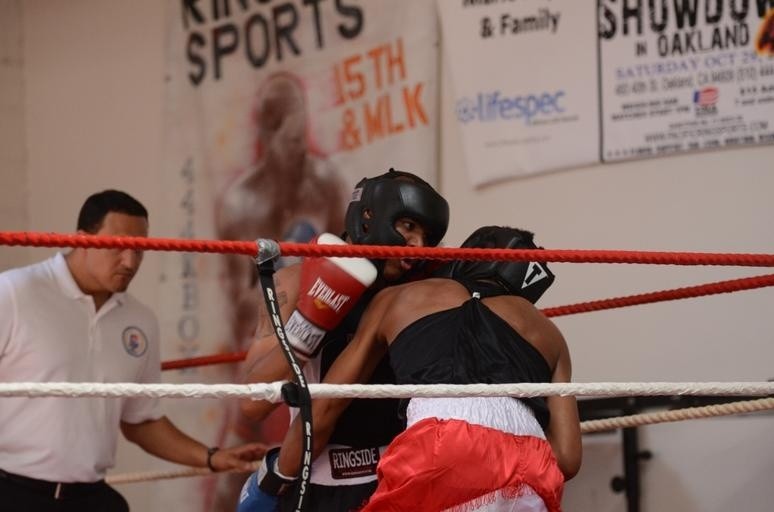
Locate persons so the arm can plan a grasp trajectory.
[0,188,267,512]
[235,226,582,512]
[241,169,450,512]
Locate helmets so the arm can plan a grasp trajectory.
[344,169,448,282]
[440,226,554,304]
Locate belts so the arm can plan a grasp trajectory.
[1,472,104,502]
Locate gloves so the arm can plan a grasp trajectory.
[282,231,379,362]
[236,446,298,511]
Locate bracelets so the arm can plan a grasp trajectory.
[207,446,220,473]
